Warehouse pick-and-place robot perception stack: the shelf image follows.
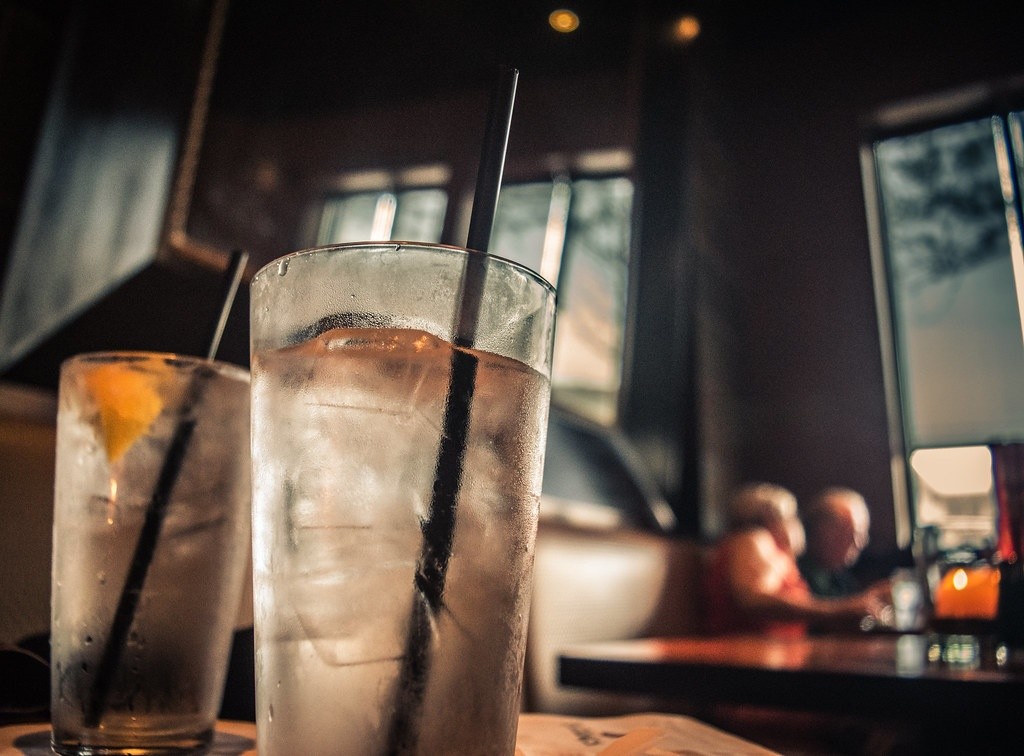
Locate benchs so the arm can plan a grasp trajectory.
[522,520,725,718]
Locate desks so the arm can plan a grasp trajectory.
[556,637,1024,756]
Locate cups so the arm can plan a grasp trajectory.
[245,236,558,756]
[48,348,251,756]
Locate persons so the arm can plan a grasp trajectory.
[703,485,895,638]
